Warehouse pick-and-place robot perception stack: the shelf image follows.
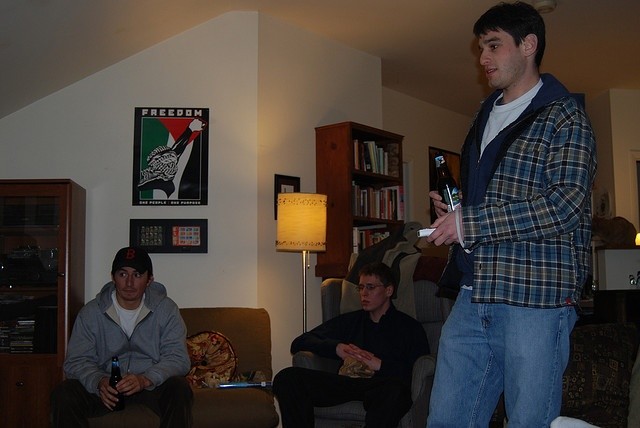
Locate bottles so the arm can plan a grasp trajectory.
[109,356,125,410]
[434,151,461,212]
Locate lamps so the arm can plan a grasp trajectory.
[274,192,329,333]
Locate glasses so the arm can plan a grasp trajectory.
[355,284,383,290]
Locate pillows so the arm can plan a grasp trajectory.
[341,253,420,317]
[186,330,238,389]
[560,323,639,428]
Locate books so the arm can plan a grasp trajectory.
[0,315,38,353]
[353,223,392,256]
[353,138,401,180]
[350,181,406,221]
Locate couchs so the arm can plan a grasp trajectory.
[87,307,281,427]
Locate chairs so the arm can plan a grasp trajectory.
[504,321,640,428]
[291,252,450,427]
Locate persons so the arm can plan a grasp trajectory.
[272,264,431,427]
[426,1,598,428]
[49,246,195,427]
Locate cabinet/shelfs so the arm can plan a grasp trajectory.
[314,121,403,276]
[0,176,86,355]
[0,355,66,428]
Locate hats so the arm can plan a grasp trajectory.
[112,247,152,274]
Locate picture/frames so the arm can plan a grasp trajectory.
[428,145,461,225]
[626,148,640,232]
[274,173,301,221]
